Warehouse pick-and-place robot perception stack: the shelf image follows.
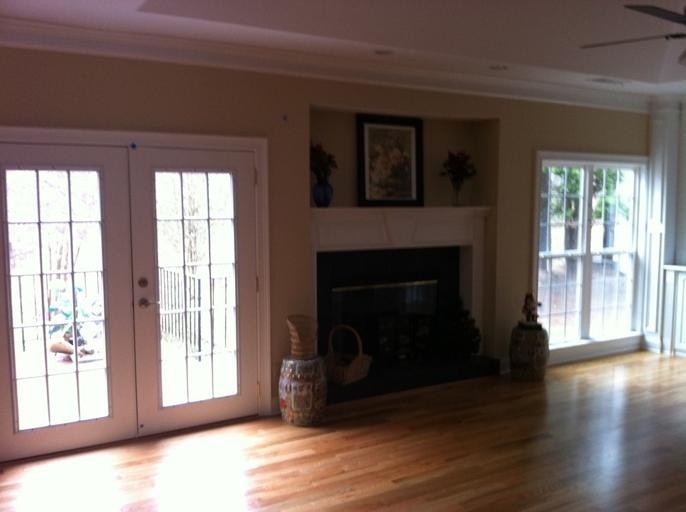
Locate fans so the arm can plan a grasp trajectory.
[580,4,686,48]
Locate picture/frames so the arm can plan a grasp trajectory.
[355,112,424,208]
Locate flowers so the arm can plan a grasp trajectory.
[439,150,479,190]
[310,143,338,176]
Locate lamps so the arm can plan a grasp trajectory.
[677,48,686,66]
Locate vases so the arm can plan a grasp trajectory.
[447,182,463,206]
[313,174,333,207]
